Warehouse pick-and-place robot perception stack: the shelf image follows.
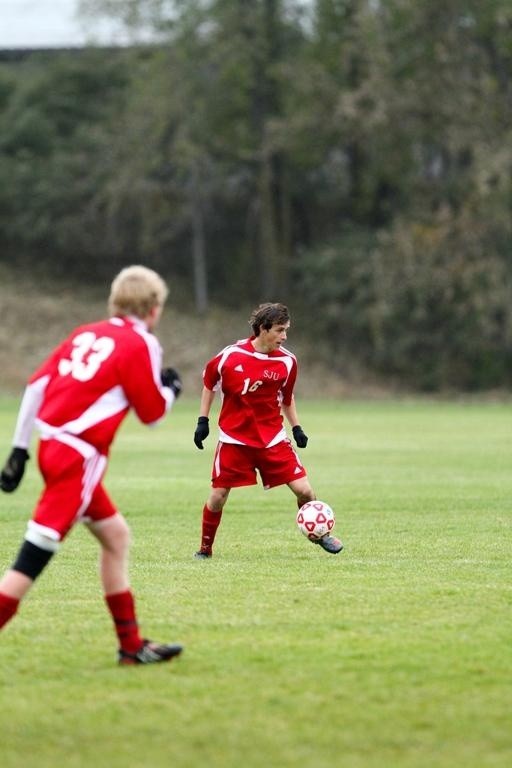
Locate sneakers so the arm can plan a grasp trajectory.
[194,548,215,559]
[117,640,184,665]
[317,533,344,554]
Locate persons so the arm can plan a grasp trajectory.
[191,300,347,561]
[0,263,183,669]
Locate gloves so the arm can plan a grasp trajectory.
[160,367,183,399]
[193,415,211,449]
[291,425,309,449]
[0,447,29,492]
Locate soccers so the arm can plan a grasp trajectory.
[296,501,335,540]
